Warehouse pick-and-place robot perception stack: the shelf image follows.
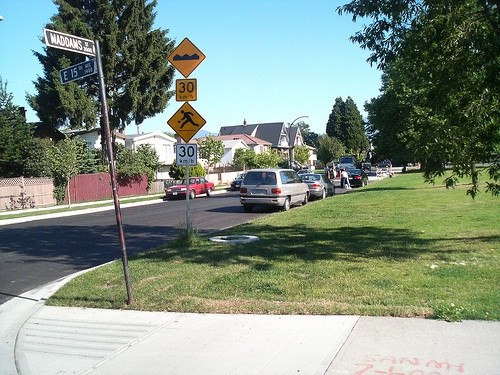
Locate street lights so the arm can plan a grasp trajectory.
[289,116,310,168]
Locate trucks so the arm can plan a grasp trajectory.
[328,156,358,178]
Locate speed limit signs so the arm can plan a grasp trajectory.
[176,79,197,101]
[176,143,197,166]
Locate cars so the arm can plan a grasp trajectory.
[166,177,214,199]
[230,172,248,190]
[341,169,369,187]
[297,169,335,199]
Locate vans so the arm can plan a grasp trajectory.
[239,169,310,211]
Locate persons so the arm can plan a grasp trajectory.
[341,169,349,189]
